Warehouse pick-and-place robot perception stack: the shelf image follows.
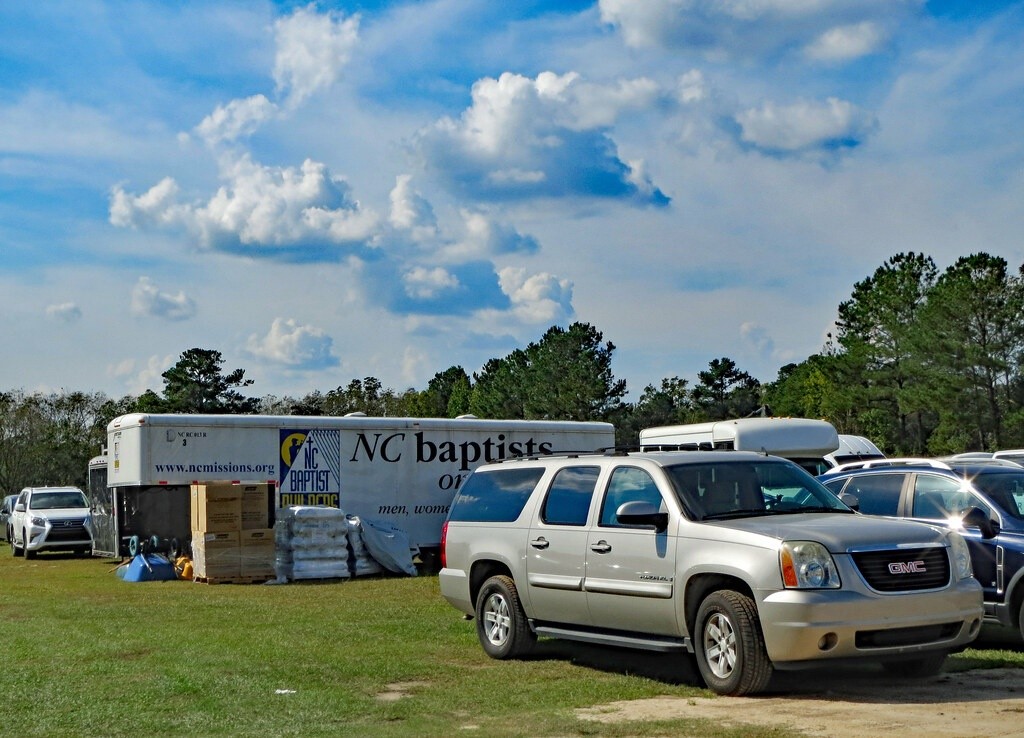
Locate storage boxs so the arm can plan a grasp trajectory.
[190,485,199,531]
[198,485,241,532]
[241,565,271,576]
[192,531,198,577]
[241,545,275,565]
[198,532,240,548]
[238,529,275,545]
[198,548,240,566]
[199,566,239,578]
[241,483,268,529]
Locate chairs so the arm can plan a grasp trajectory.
[916,491,945,517]
[545,487,578,522]
[702,482,743,517]
[610,475,656,525]
[850,488,874,514]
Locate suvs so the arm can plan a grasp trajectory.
[638,417,1024,646]
[0,484,97,560]
[438,445,985,695]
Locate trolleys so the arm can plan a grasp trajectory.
[108,534,182,583]
